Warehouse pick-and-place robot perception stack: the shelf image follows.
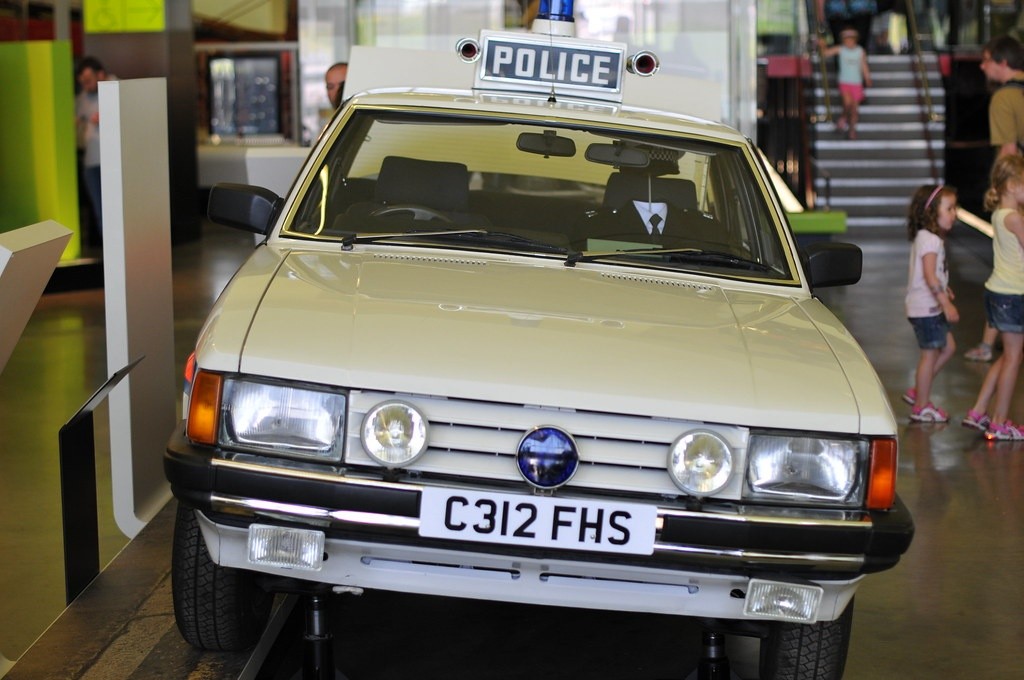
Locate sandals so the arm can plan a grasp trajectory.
[984,419,1024,440]
[910,401,950,422]
[902,388,934,407]
[961,409,992,430]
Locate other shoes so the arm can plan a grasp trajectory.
[849,129,856,139]
[965,348,993,361]
[836,117,847,129]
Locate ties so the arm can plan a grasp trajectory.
[649,214,662,236]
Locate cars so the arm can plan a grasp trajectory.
[160,79,918,680]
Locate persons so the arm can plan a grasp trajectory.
[962,34,1024,441]
[325,63,347,109]
[901,183,960,424]
[74,58,119,236]
[815,0,879,140]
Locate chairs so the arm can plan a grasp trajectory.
[595,168,697,242]
[374,156,470,219]
